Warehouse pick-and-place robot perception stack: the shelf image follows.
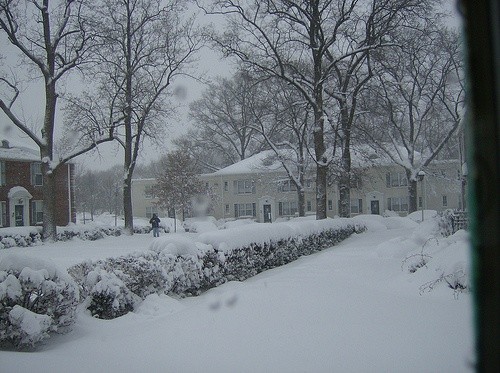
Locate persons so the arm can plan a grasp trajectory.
[150,213,160,238]
